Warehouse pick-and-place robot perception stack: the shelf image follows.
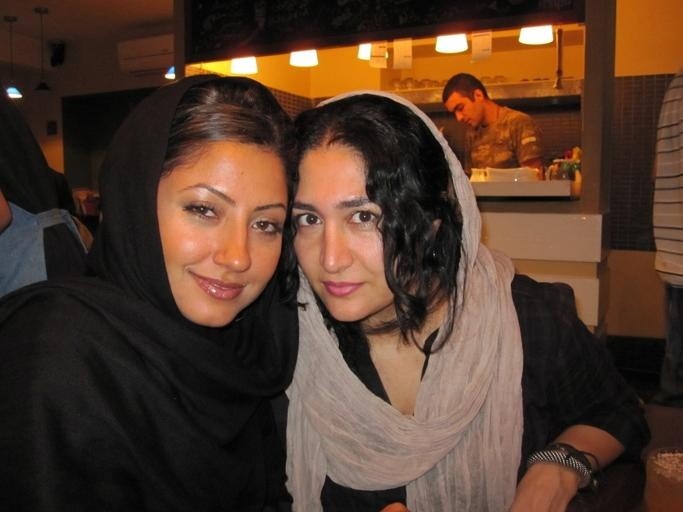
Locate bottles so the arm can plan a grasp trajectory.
[547,158,582,199]
[469,168,486,181]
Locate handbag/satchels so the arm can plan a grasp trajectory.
[567,462,646,512]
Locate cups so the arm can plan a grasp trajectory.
[390,74,509,91]
